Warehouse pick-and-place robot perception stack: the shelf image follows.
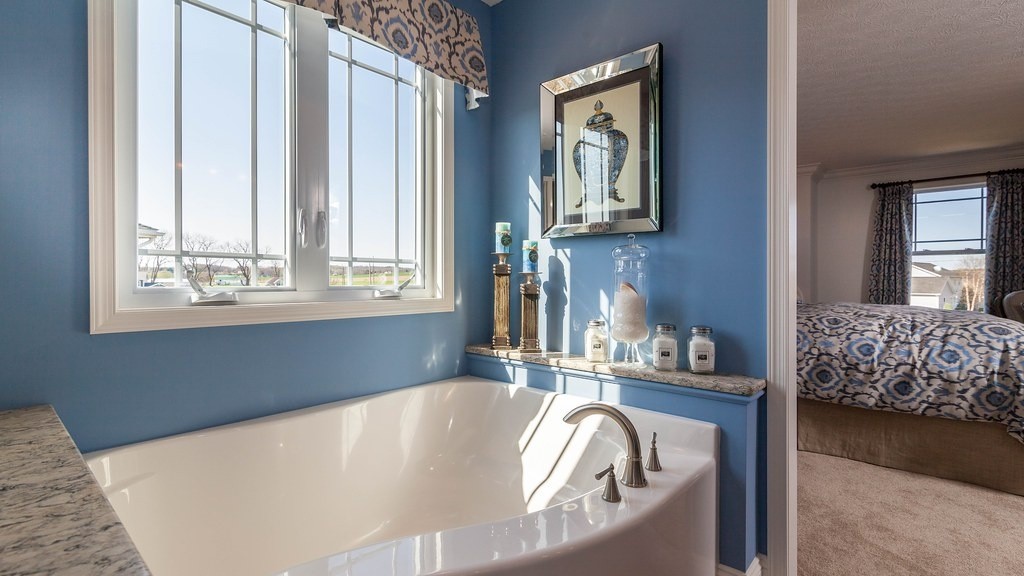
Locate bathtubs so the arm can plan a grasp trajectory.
[82,375,720,576]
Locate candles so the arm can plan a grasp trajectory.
[495,221,510,254]
[522,241,539,272]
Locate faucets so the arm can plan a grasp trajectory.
[563,404,648,488]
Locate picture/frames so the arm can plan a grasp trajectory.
[540,43,662,239]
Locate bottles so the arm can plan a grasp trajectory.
[652,324,679,372]
[686,326,715,374]
[584,320,608,362]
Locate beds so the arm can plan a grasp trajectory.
[796,301,1024,497]
